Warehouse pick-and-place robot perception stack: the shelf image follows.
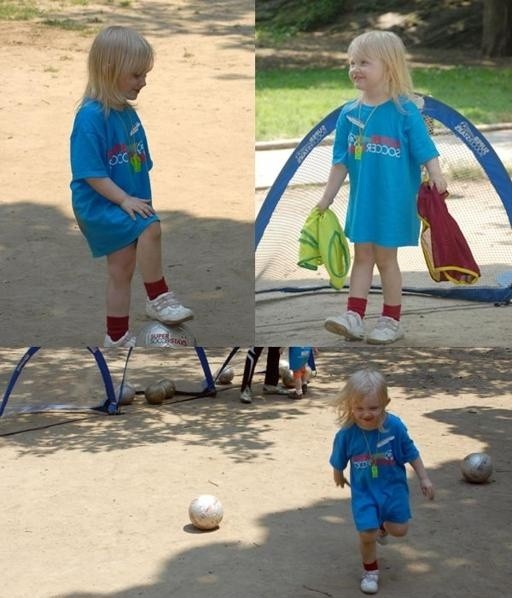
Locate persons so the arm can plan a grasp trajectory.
[68,24,197,347]
[314,27,450,347]
[278,347,319,400]
[329,368,436,595]
[239,347,290,404]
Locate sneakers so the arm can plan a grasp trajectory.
[263,384,311,398]
[376,530,388,546]
[360,560,380,594]
[240,388,252,403]
[146,291,192,325]
[104,330,136,347]
[324,310,406,344]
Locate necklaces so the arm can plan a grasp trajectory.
[358,429,385,480]
[108,103,144,174]
[352,96,379,159]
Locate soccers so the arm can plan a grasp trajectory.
[278,360,311,386]
[190,495,224,529]
[115,378,175,404]
[216,366,234,383]
[461,453,494,483]
[135,322,197,348]
